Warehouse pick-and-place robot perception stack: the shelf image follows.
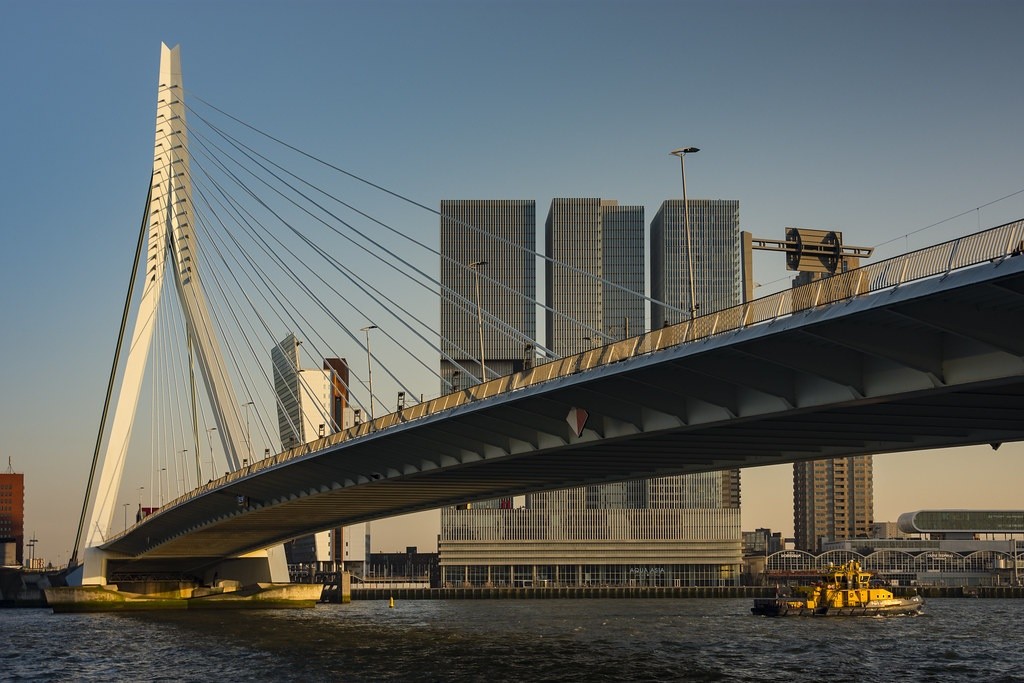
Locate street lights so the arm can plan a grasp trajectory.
[136,486,145,523]
[205,426,218,482]
[122,502,130,535]
[179,449,188,496]
[669,145,703,319]
[360,324,377,420]
[157,466,167,512]
[241,401,254,466]
[470,260,486,384]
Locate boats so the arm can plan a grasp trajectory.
[750,559,926,618]
